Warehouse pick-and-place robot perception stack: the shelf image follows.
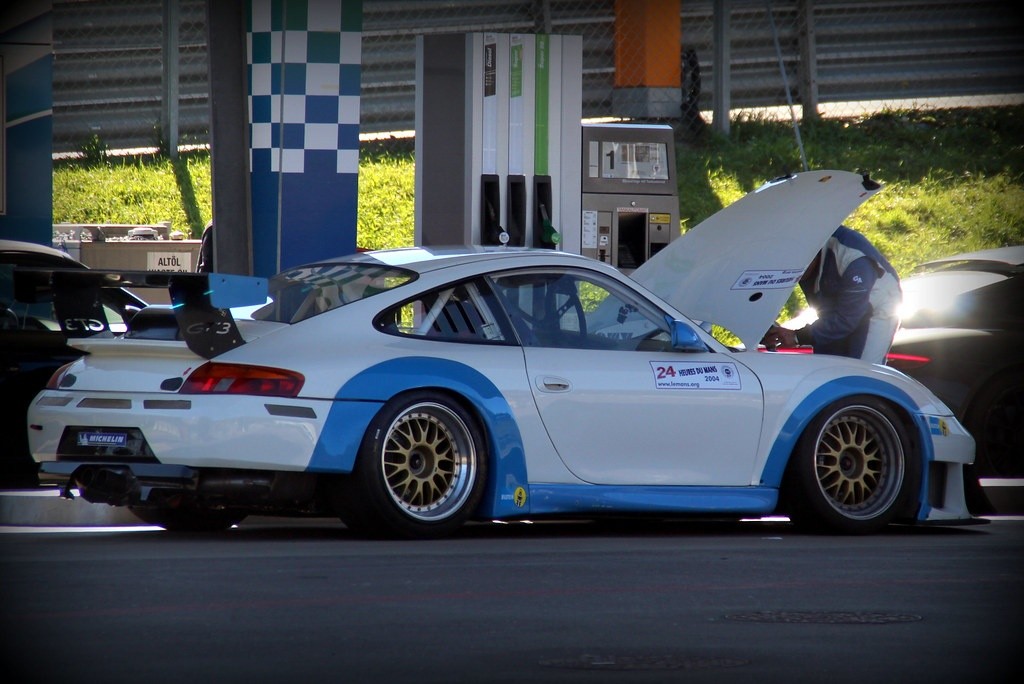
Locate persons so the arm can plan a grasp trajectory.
[761,225,904,366]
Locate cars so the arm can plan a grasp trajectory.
[0,239,150,446]
[778,244,1023,476]
[24,168,978,541]
